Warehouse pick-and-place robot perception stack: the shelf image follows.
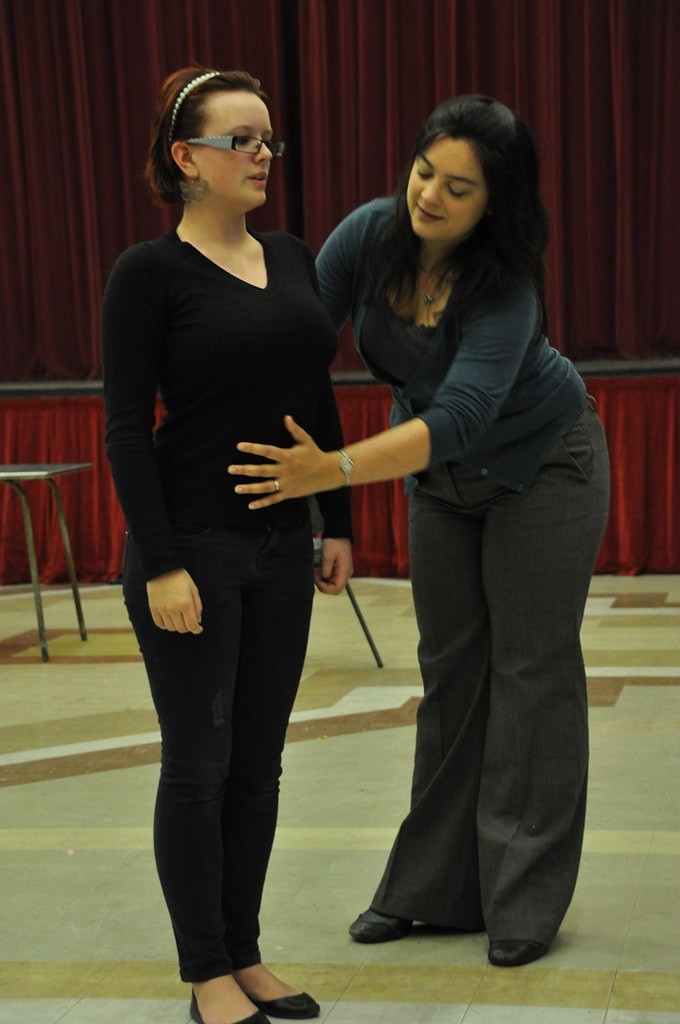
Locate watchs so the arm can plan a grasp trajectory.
[339,449,355,487]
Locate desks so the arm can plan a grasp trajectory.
[0,463,94,663]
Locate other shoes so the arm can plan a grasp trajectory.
[487,940,548,967]
[348,908,415,941]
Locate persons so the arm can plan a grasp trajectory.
[227,96,610,970]
[98,66,352,1024]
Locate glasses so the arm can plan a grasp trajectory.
[187,134,286,158]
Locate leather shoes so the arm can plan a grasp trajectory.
[189,979,320,1024]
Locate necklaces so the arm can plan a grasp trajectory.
[415,276,448,305]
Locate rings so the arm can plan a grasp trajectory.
[274,480,279,492]
